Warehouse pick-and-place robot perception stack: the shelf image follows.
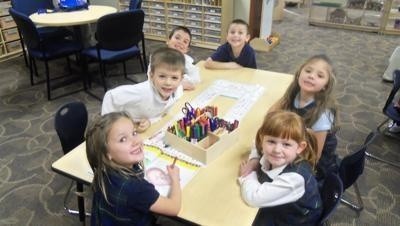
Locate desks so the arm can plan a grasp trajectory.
[29,4,118,48]
[51,60,296,226]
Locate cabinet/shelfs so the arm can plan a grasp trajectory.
[117,0,234,51]
[0,0,28,64]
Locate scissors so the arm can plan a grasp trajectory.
[181,102,195,116]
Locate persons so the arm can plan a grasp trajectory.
[99,46,185,132]
[204,19,258,70]
[146,26,202,91]
[71,111,182,226]
[238,109,325,226]
[268,53,340,175]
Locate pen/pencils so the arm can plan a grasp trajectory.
[173,105,239,141]
[171,157,177,169]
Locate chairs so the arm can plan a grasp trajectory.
[8,6,87,101]
[54,102,93,223]
[362,69,400,168]
[127,1,144,47]
[318,171,343,226]
[84,8,149,102]
[7,0,56,68]
[319,131,377,211]
